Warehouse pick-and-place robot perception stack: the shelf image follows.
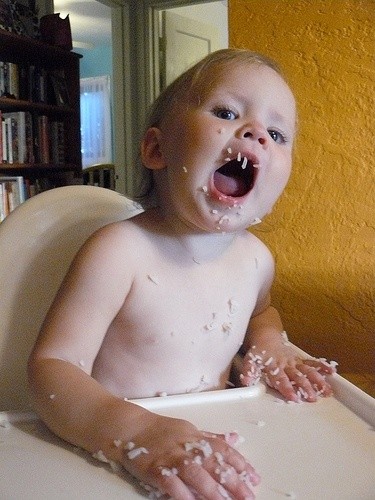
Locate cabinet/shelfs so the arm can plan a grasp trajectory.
[0,76,78,186]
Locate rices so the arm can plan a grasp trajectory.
[47,148,340,500]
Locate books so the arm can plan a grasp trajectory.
[0,56,82,229]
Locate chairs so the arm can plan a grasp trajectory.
[3,182,143,411]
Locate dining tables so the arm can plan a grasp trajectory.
[1,368,374,499]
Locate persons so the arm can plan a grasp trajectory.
[25,47,337,500]
